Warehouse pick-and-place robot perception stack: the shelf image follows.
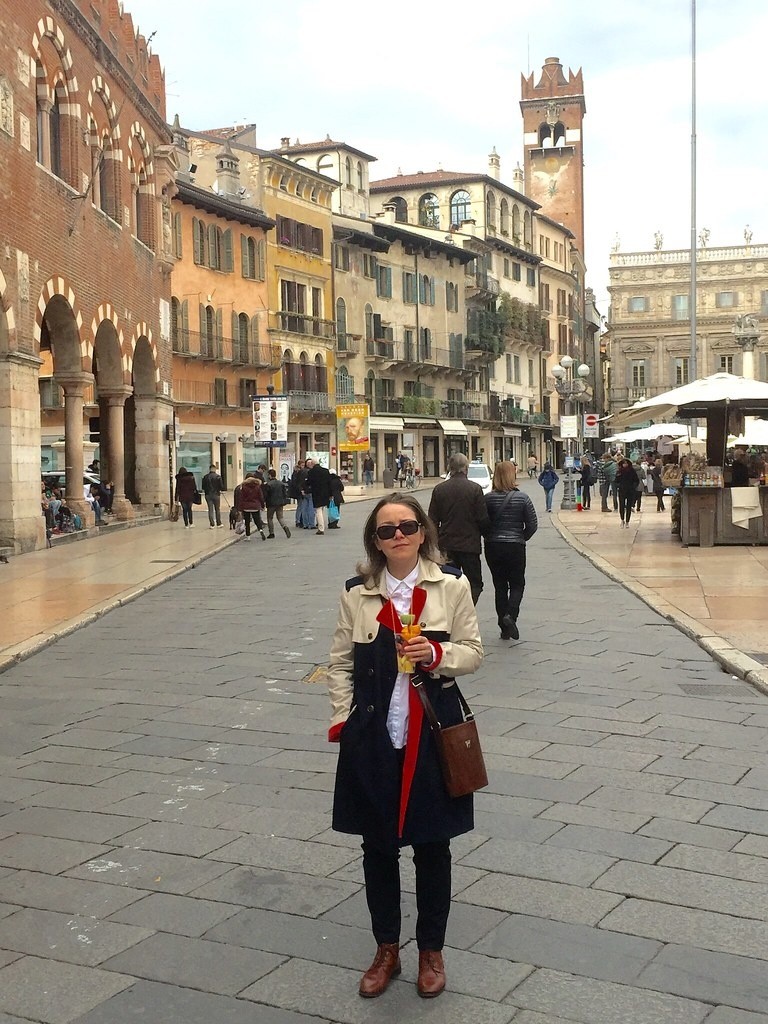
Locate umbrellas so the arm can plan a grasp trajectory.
[596,373,768,474]
[601,424,707,444]
[727,419,768,447]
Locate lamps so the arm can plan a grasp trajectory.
[444,235,454,245]
[238,432,251,442]
[215,431,228,443]
[188,163,198,174]
[209,179,219,196]
[236,186,246,196]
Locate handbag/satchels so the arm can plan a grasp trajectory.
[434,719,489,797]
[235,522,245,534]
[341,495,344,503]
[287,487,296,499]
[194,491,201,504]
[328,500,340,523]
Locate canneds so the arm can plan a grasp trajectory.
[691,472,719,486]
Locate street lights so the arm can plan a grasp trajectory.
[551,355,591,510]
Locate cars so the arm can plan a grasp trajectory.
[41,470,102,504]
[439,463,494,496]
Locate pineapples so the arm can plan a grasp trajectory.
[396,654,415,672]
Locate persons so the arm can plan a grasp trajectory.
[484,461,538,639]
[363,454,375,486]
[476,448,559,512]
[282,464,291,498]
[41,480,71,534]
[254,402,277,439]
[427,452,492,607]
[202,465,223,528]
[84,481,114,526]
[290,458,345,534]
[88,460,100,474]
[725,447,768,487]
[345,416,364,443]
[327,493,485,995]
[394,451,411,481]
[561,449,687,528]
[175,467,199,528]
[239,464,291,540]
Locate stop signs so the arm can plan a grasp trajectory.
[586,416,597,426]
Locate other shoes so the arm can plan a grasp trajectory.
[503,614,519,640]
[262,534,266,540]
[243,536,250,540]
[316,530,325,535]
[501,630,510,639]
[267,534,275,539]
[284,526,291,538]
[602,508,611,512]
[190,524,195,527]
[185,526,188,528]
[217,524,224,528]
[210,525,215,529]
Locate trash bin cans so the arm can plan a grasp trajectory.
[383,467,394,489]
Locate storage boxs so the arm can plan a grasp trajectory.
[704,466,723,477]
[662,479,682,487]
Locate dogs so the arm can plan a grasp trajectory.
[229,507,242,530]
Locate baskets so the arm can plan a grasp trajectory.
[662,478,684,487]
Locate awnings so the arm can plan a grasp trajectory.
[440,420,468,435]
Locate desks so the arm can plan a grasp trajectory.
[677,486,768,549]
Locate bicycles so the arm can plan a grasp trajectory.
[528,462,539,478]
[406,468,423,489]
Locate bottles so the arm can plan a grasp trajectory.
[760,471,765,485]
[685,472,722,486]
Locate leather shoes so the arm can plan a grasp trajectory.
[418,952,446,999]
[359,943,401,997]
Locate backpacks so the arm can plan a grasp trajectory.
[586,467,598,485]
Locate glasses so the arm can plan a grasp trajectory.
[375,521,421,540]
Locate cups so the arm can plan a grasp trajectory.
[395,633,418,673]
